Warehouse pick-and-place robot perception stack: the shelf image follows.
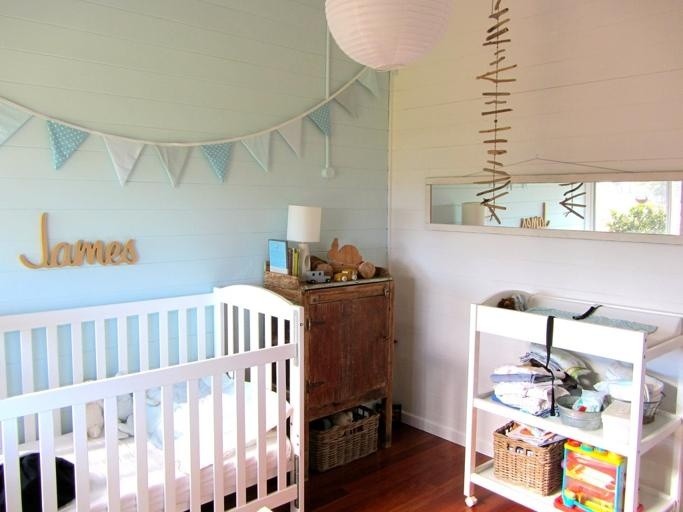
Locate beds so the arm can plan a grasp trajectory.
[1,286,307,512]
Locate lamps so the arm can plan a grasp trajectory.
[286,203,322,281]
[324,1,453,74]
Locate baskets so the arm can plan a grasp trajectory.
[492,420,568,496]
[309,405,380,473]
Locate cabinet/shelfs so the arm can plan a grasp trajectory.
[464,290,682,512]
[264,265,396,451]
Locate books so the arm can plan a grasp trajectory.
[492,422,560,493]
[267,236,299,282]
